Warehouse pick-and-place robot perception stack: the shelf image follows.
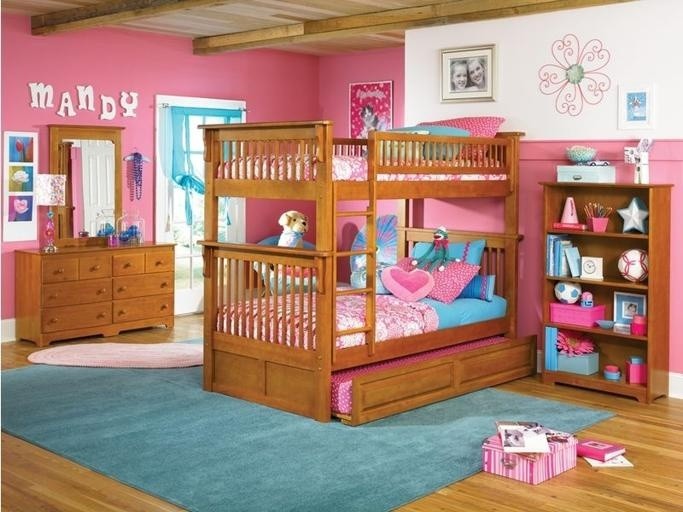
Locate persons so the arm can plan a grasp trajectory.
[625,304,637,316]
[466,57,486,89]
[451,61,479,90]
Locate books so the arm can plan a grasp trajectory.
[583,454,634,468]
[545,234,582,278]
[574,437,627,462]
[492,420,567,453]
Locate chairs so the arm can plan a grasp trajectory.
[250,235,317,296]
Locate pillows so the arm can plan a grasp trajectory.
[363,116,504,162]
[350,241,496,305]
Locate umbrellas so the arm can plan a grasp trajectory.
[348,212,399,271]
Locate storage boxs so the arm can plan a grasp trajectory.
[548,300,606,329]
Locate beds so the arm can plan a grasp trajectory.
[196,120,537,427]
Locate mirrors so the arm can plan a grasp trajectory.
[43,122,127,248]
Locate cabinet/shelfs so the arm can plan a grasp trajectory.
[15,242,179,347]
[535,180,675,405]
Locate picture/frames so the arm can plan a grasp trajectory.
[439,43,496,105]
[612,290,647,324]
[617,84,654,131]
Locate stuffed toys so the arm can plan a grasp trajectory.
[412,224,450,273]
[277,209,310,270]
